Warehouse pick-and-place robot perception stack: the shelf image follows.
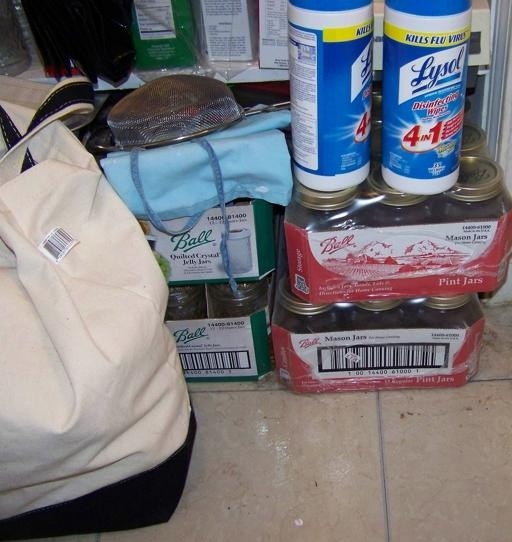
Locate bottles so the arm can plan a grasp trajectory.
[460,121,488,155]
[417,294,482,328]
[276,276,346,333]
[433,154,512,222]
[332,300,354,330]
[345,300,411,329]
[165,284,207,320]
[404,297,428,328]
[367,163,432,227]
[211,281,262,315]
[285,182,370,232]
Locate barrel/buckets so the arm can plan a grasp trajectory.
[221,229,252,274]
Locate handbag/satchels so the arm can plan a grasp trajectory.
[2,72,197,539]
